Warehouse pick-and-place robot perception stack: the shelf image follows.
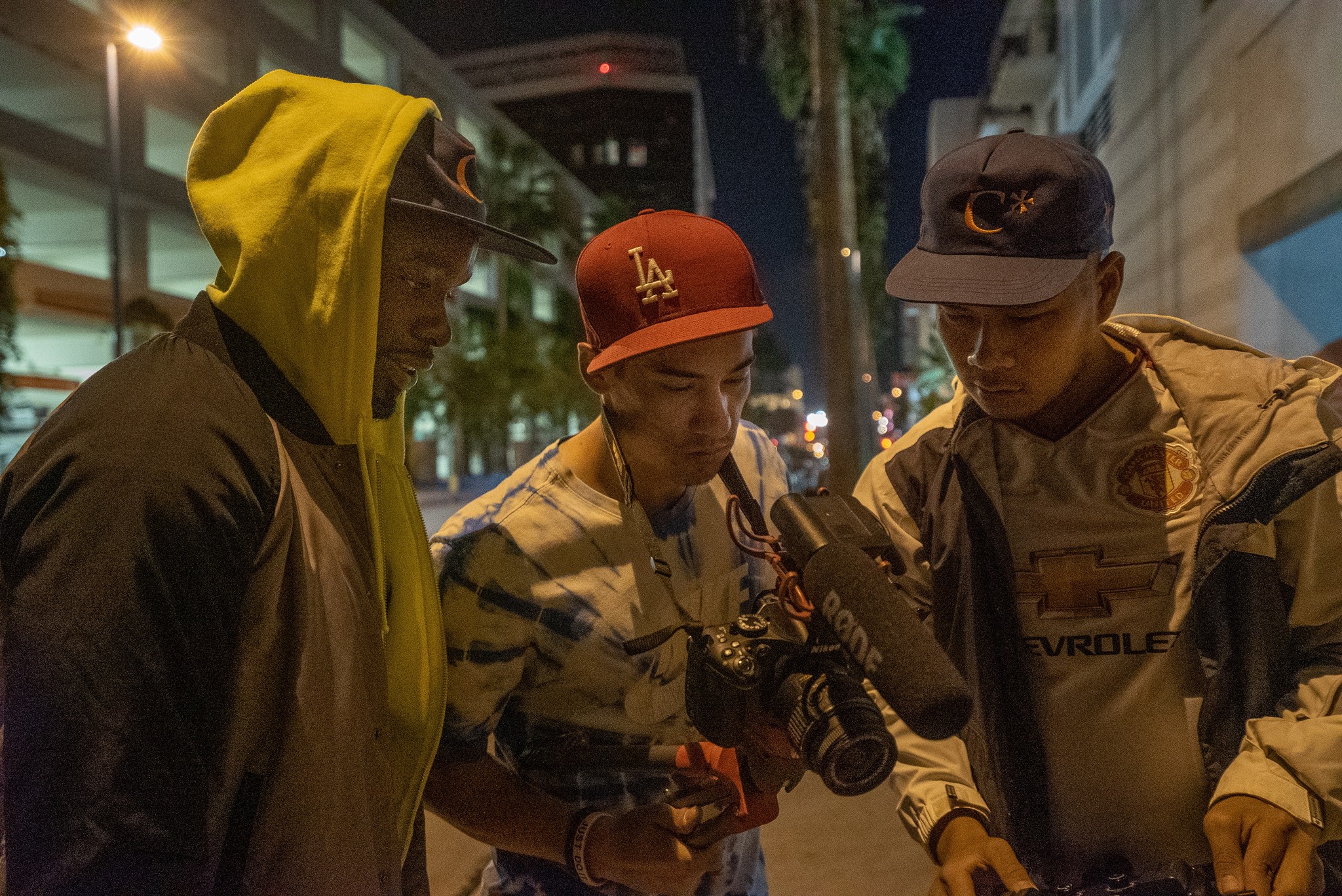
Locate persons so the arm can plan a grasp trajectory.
[851,128,1342,896]
[421,209,791,896]
[0,69,558,896]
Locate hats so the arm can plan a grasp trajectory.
[885,127,1116,304]
[575,208,773,374]
[385,113,558,265]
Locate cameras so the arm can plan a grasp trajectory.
[674,500,908,796]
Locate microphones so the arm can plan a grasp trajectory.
[797,541,972,742]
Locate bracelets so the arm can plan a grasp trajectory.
[573,812,616,887]
[564,807,596,884]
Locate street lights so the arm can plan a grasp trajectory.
[105,26,163,357]
